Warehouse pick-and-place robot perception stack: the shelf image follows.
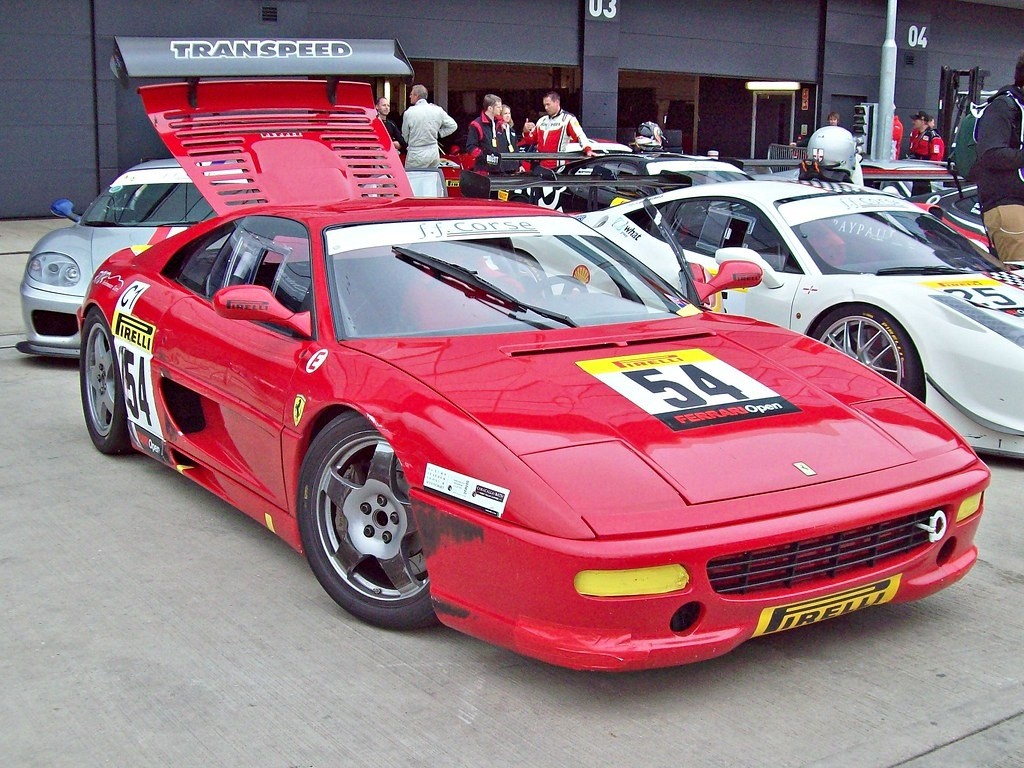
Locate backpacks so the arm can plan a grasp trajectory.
[955,91,1024,182]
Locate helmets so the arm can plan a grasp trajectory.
[636,121,668,142]
[807,127,857,178]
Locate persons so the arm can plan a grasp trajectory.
[828,111,840,126]
[375,85,457,170]
[466,90,598,172]
[976,51,1024,262]
[908,111,945,161]
[890,102,903,159]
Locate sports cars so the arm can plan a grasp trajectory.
[725,157,997,258]
[76,34,990,675]
[458,144,1023,460]
[15,155,254,361]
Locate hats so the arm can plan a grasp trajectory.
[910,111,929,122]
